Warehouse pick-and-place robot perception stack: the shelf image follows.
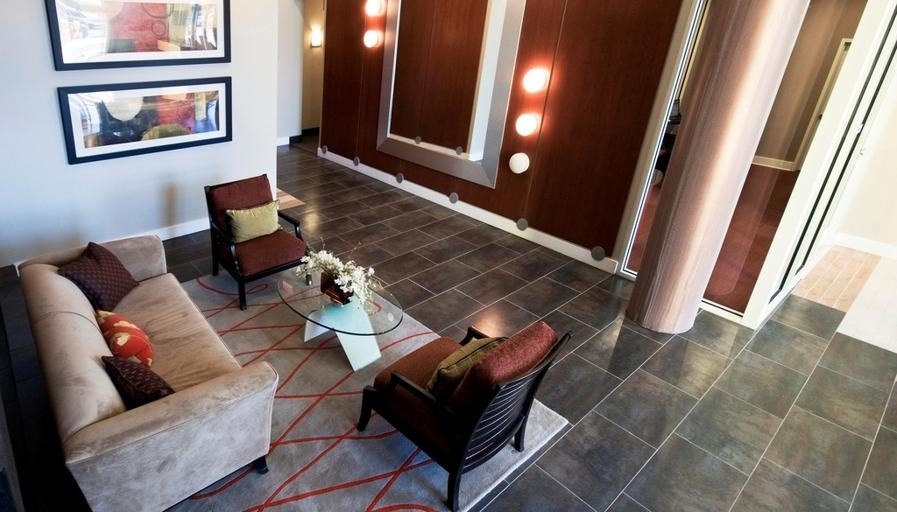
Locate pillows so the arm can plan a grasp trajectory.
[57,241,139,311]
[226,199,283,243]
[99,354,174,406]
[426,335,509,396]
[95,309,154,369]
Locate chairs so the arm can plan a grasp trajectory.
[204,174,313,310]
[356,324,572,512]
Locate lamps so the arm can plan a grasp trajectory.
[509,153,530,175]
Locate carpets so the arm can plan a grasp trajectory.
[277,187,305,210]
[167,258,572,512]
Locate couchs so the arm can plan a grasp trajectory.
[17,237,278,511]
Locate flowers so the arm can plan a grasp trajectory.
[296,249,374,310]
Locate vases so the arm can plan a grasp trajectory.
[320,272,353,304]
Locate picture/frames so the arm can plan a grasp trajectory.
[57,76,232,164]
[45,0,231,70]
